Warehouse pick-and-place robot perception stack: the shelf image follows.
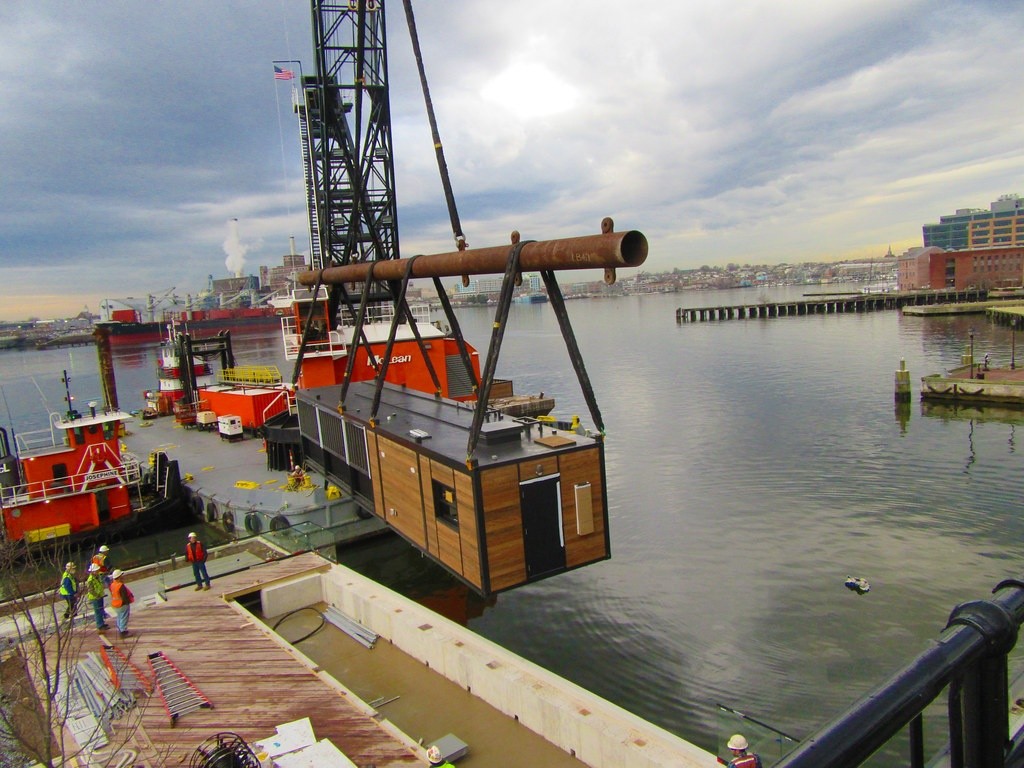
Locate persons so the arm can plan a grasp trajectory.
[727,735,763,768]
[292,465,305,481]
[87,563,110,631]
[86,545,110,597]
[185,532,210,591]
[109,570,133,638]
[59,562,79,620]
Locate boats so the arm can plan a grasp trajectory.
[93,284,348,350]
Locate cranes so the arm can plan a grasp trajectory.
[294,2,485,410]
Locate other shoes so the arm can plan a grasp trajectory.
[195,586,202,591]
[64,613,77,618]
[99,625,111,630]
[120,631,134,639]
[203,585,211,590]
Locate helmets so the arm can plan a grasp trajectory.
[65,562,76,569]
[99,545,110,552]
[112,570,123,579]
[187,532,196,537]
[88,563,100,571]
